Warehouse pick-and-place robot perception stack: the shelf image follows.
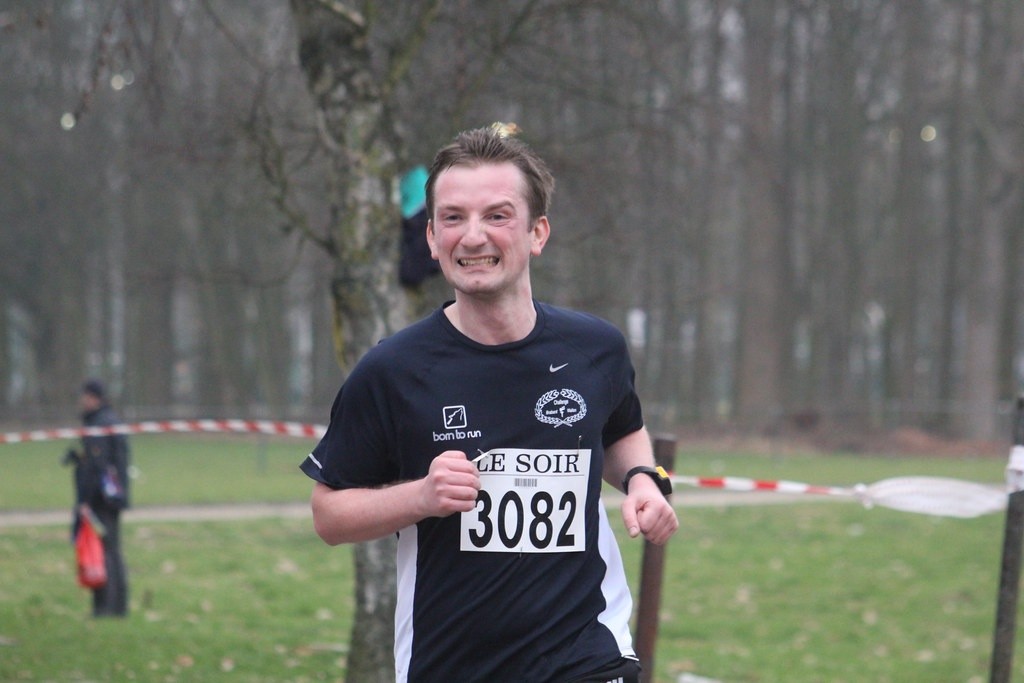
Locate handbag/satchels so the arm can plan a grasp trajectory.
[76,517,105,587]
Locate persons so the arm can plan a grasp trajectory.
[299,126,679,683]
[62,382,133,618]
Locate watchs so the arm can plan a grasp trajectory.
[621,465,673,496]
